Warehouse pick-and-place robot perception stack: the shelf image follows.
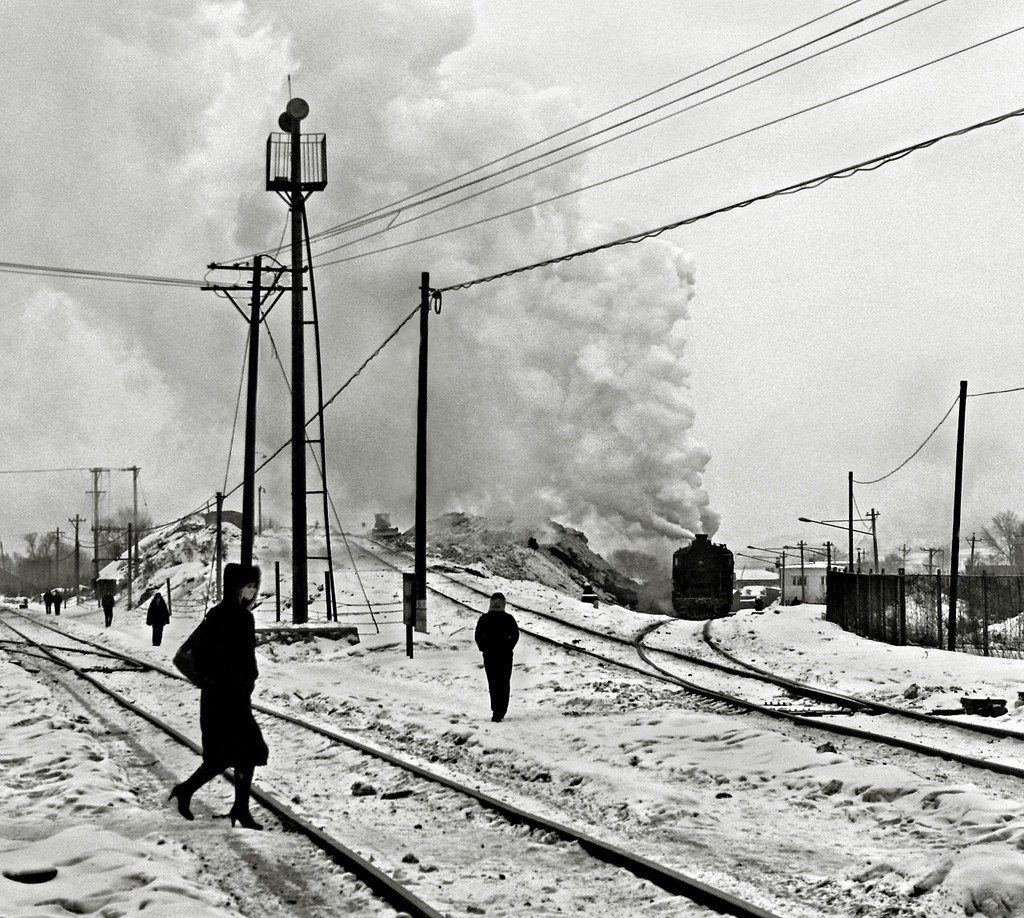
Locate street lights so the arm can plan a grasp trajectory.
[798,517,880,574]
[783,541,832,573]
[747,545,806,604]
[737,552,786,606]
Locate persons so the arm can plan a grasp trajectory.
[44,588,62,615]
[168,562,269,831]
[101,588,114,627]
[475,593,519,724]
[146,592,170,646]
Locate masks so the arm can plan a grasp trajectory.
[240,587,256,600]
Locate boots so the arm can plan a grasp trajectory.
[230,791,263,830]
[168,768,208,821]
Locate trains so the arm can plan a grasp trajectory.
[671,533,736,621]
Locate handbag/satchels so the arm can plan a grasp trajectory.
[173,616,211,685]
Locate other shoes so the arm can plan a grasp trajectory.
[492,711,505,722]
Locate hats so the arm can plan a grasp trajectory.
[491,593,505,600]
